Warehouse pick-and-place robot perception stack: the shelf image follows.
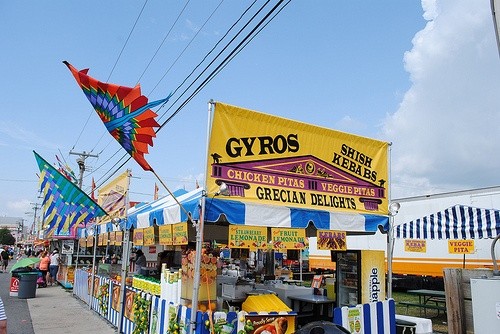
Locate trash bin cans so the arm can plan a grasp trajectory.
[18,272,38,299]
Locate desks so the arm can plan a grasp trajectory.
[407,289,447,319]
[287,294,336,328]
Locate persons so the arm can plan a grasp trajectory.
[0,296,7,334]
[0,244,63,288]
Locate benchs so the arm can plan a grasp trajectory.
[399,301,446,319]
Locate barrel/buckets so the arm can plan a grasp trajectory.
[18,274,37,298]
[326,278,336,300]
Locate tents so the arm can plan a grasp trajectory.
[53,185,500,334]
